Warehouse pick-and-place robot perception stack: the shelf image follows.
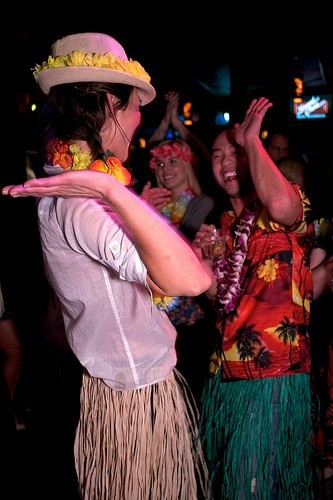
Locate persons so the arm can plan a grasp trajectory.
[2,35,211,500]
[0,287,84,500]
[120,89,332,500]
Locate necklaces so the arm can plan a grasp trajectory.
[154,186,196,311]
[215,201,264,313]
[47,136,131,186]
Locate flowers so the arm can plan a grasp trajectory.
[30,48,151,83]
[149,142,194,169]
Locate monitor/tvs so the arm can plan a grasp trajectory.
[290,94,332,120]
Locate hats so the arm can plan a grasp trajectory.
[32,33,156,106]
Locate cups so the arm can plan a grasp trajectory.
[204,229,232,261]
